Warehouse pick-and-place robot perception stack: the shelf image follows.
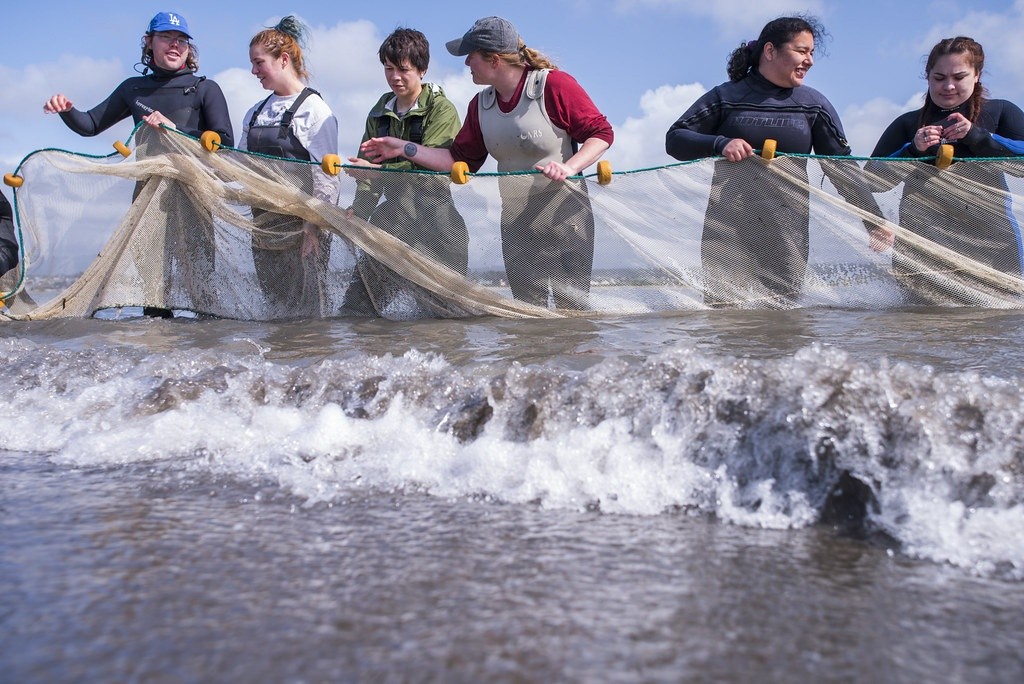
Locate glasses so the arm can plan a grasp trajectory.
[152,34,189,46]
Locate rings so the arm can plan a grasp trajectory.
[923,131,927,136]
[957,128,960,133]
[925,137,927,143]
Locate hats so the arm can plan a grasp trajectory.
[146,12,194,40]
[445,16,519,56]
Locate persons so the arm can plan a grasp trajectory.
[866,37,1024,308]
[0,189,19,309]
[214,18,341,318]
[43,12,234,316]
[665,17,891,309]
[362,16,614,311]
[345,27,469,317]
